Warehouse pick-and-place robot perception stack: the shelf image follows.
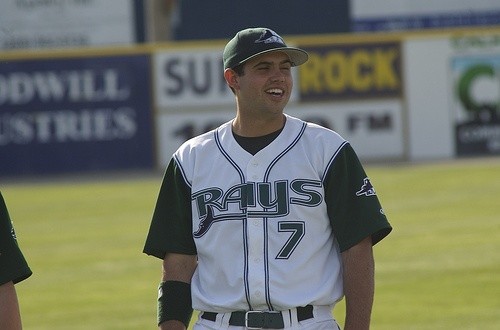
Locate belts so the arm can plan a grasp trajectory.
[201,304,314,330]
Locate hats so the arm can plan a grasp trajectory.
[222,27,310,72]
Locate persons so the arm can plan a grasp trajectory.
[143,28,393,329]
[0,191,33,330]
